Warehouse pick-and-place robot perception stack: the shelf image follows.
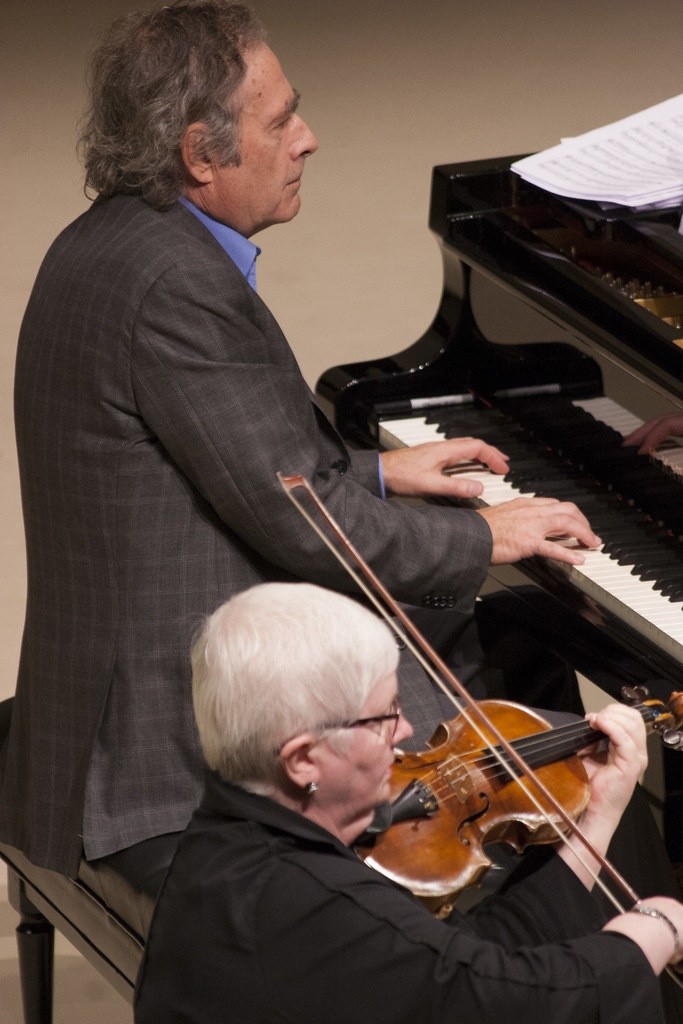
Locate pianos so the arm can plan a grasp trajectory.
[310,146,683,851]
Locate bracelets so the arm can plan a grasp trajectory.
[631,907,680,954]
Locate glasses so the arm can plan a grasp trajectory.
[272,701,403,755]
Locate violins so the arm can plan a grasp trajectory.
[354,688,683,916]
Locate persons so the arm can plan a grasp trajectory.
[0,1,601,878]
[133,582,683,1024]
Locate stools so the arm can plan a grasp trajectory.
[1,695,185,1024]
